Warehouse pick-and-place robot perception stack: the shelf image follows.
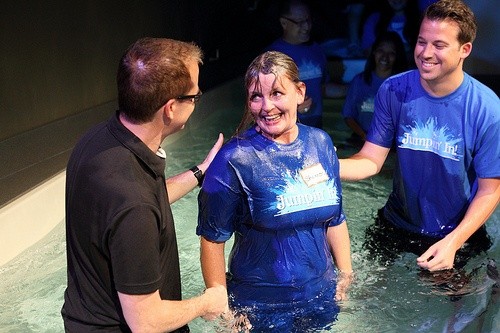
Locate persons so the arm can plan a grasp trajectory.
[338,0,500,276]
[265,0,415,148]
[196,51,353,333]
[61,38,227,333]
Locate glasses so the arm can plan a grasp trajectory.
[178,90,203,103]
[282,17,311,26]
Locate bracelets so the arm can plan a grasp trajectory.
[189,166,204,186]
